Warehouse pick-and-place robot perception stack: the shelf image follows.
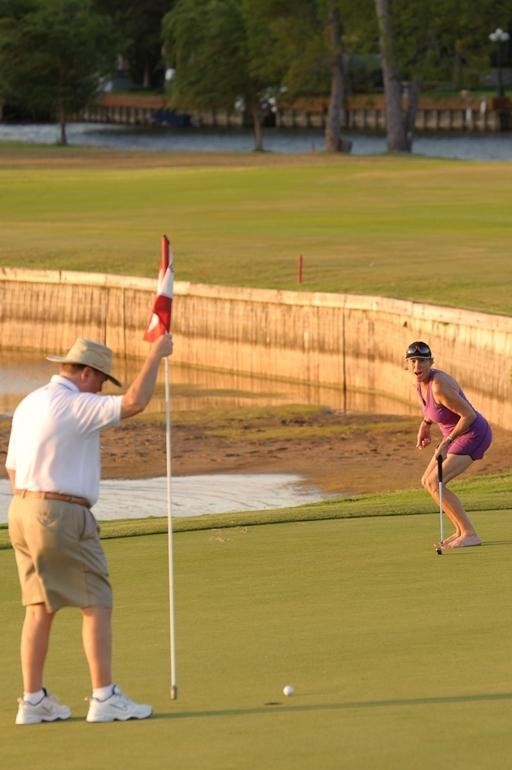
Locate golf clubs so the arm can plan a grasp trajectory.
[434,446,444,554]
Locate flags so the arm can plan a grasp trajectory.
[137,231,177,342]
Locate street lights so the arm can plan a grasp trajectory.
[488,28,510,97]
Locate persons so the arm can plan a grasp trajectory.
[405,338,494,550]
[3,335,172,723]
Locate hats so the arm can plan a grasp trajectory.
[44,334,124,389]
[402,340,432,361]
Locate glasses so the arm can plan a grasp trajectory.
[405,344,430,356]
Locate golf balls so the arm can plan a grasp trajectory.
[284,686,294,697]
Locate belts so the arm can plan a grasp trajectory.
[10,487,94,509]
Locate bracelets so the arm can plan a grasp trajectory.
[447,436,453,444]
[422,420,432,426]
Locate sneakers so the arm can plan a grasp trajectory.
[82,682,156,724]
[13,686,74,727]
